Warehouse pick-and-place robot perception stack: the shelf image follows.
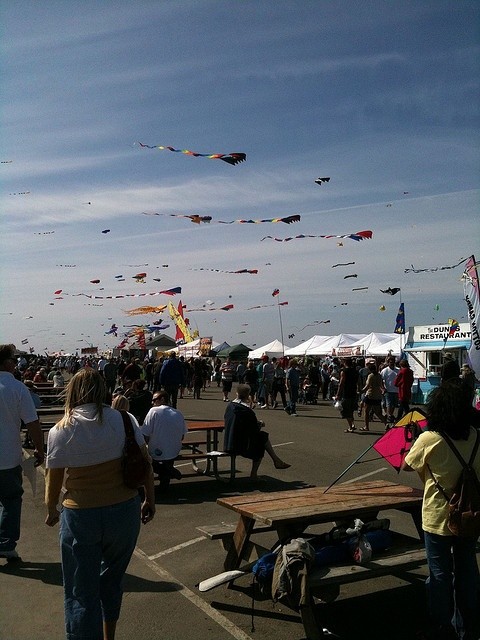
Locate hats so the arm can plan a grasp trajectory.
[442,353,451,358]
[462,363,472,371]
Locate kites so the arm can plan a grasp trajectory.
[54,289,64,294]
[113,274,124,278]
[257,230,375,252]
[297,318,334,335]
[120,303,169,316]
[104,322,119,337]
[138,208,213,231]
[217,211,305,232]
[88,279,102,283]
[130,323,171,332]
[131,271,150,278]
[384,287,401,296]
[188,265,261,275]
[247,300,290,313]
[345,274,358,280]
[332,261,357,270]
[351,287,370,293]
[130,140,248,168]
[186,304,234,317]
[153,278,161,283]
[117,277,125,282]
[314,175,331,186]
[72,283,184,303]
[102,229,112,236]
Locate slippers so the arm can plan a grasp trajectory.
[344,429,354,433]
[360,427,369,431]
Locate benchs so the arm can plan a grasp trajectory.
[38,420,58,444]
[179,439,220,450]
[174,450,232,478]
[300,545,433,586]
[195,521,293,551]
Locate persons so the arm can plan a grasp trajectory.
[0,340,47,565]
[400,379,480,640]
[219,356,234,401]
[66,351,143,390]
[22,376,43,409]
[284,357,302,415]
[236,354,289,411]
[111,395,132,411]
[303,358,339,405]
[334,355,359,432]
[461,363,479,393]
[144,352,213,410]
[140,388,188,493]
[381,356,401,419]
[378,363,390,419]
[113,379,131,398]
[359,356,377,418]
[439,352,460,384]
[15,348,66,388]
[357,363,388,430]
[125,380,154,426]
[392,358,415,419]
[222,382,291,486]
[43,367,163,640]
[109,394,151,464]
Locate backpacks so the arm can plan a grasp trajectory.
[426,430,480,538]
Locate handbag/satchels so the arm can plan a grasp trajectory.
[117,436,146,489]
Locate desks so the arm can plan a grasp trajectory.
[34,406,65,415]
[36,386,66,390]
[176,419,228,479]
[218,479,427,572]
[38,395,66,400]
[34,381,54,386]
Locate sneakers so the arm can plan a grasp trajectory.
[273,401,278,409]
[291,413,298,417]
[0,549,18,559]
[274,462,292,469]
[261,404,269,409]
[284,407,291,415]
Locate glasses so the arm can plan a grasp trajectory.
[9,358,18,364]
[152,398,159,402]
